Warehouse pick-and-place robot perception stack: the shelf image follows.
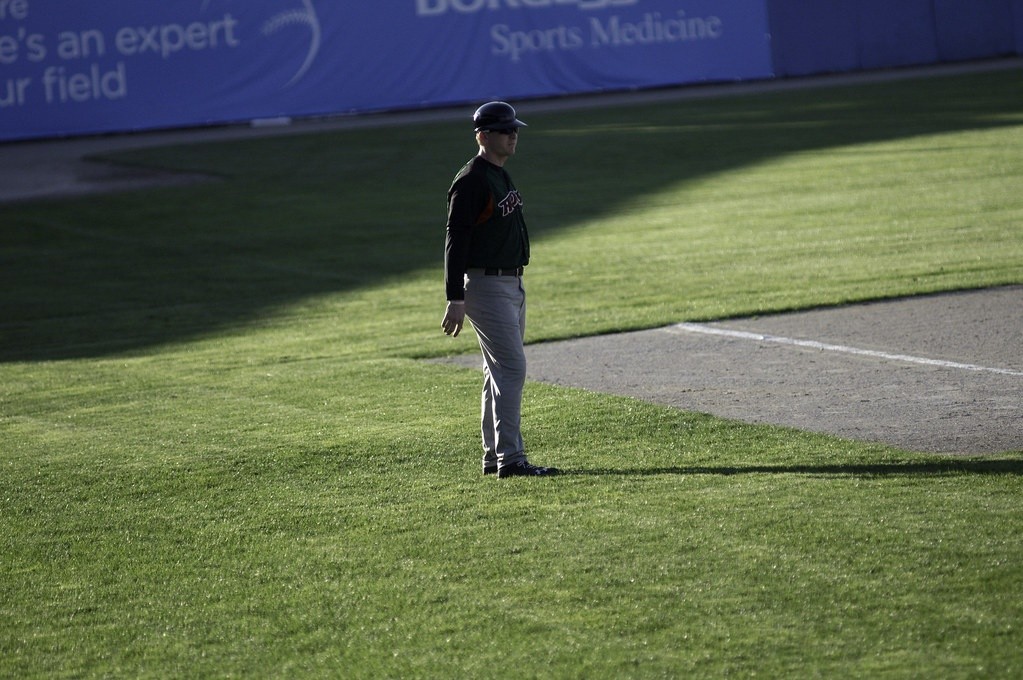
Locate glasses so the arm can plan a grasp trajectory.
[481,127,520,135]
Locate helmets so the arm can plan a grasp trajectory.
[473,102,528,132]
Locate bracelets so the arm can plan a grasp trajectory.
[449,301,465,304]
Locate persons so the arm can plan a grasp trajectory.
[442,102,566,478]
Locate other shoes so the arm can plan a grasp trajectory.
[498,461,559,479]
[484,466,498,475]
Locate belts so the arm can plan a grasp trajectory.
[484,267,524,276]
